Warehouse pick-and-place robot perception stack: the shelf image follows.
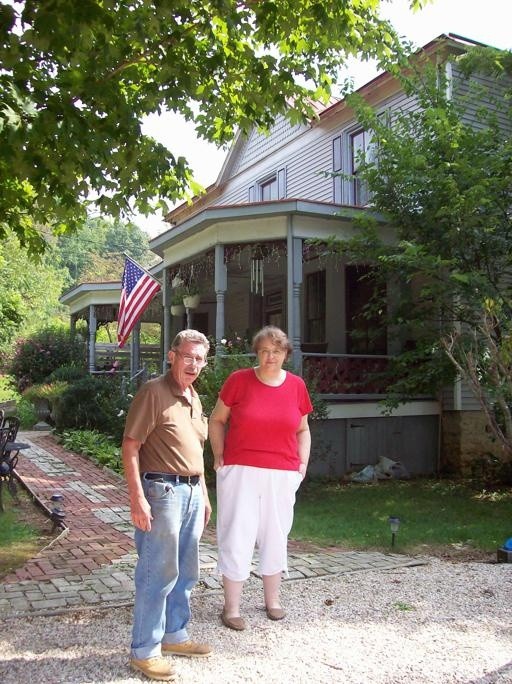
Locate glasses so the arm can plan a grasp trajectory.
[175,349,208,367]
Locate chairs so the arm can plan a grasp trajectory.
[0,428,12,512]
[0,417,21,442]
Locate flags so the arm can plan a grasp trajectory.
[114,250,161,348]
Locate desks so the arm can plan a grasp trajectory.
[0,442,31,483]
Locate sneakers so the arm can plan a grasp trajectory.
[266,602,285,620]
[161,640,212,657]
[222,609,244,630]
[129,656,179,680]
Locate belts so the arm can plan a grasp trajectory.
[145,473,199,485]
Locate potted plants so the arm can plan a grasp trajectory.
[170,298,186,317]
[182,286,202,310]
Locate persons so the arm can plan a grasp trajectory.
[207,322,314,633]
[119,327,215,682]
